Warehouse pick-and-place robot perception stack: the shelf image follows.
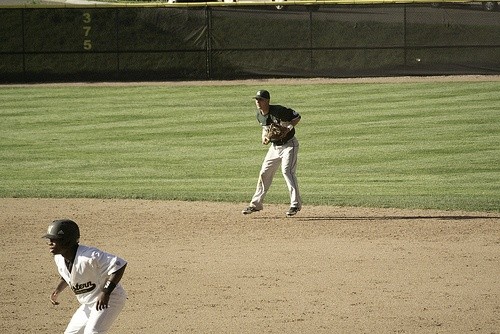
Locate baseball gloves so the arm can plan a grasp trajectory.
[265,122,287,141]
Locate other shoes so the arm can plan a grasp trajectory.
[242,206,259,215]
[287,206,302,216]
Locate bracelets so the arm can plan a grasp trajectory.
[102,278,116,296]
[287,125,293,131]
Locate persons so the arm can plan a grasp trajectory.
[40,219,127,334]
[242,90,302,216]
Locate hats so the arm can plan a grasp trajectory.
[41,219,80,241]
[252,90,270,100]
